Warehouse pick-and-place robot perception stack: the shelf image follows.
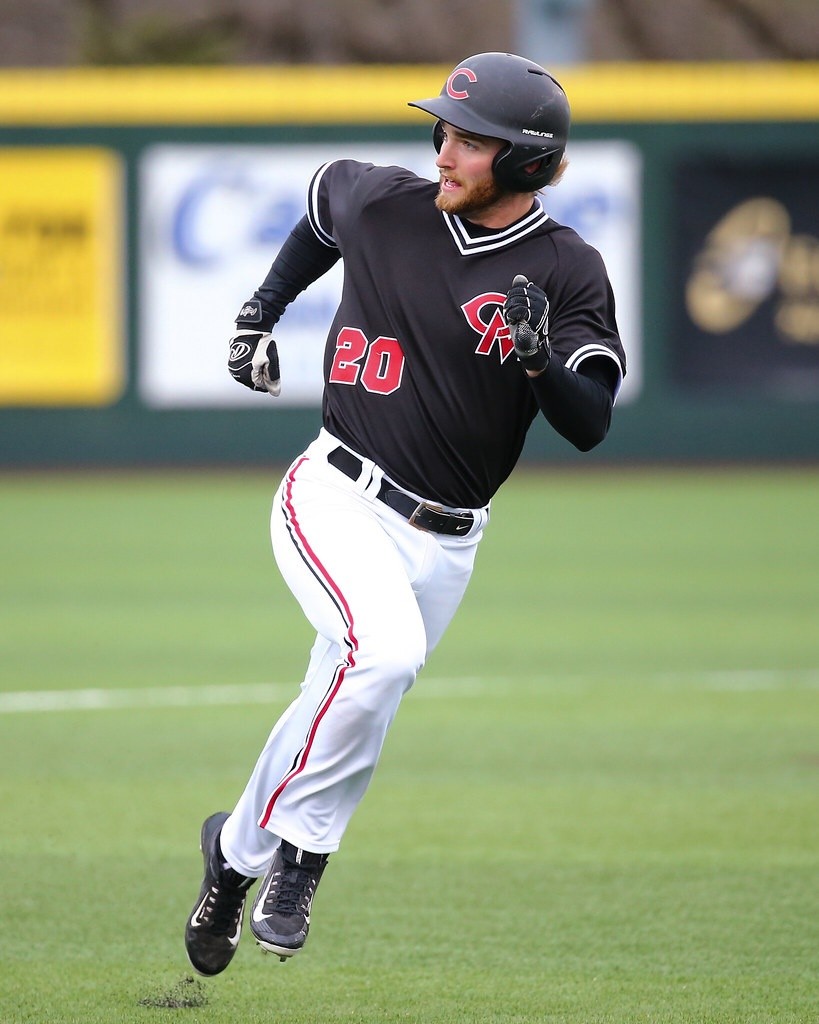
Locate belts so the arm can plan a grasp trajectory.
[328,446,474,536]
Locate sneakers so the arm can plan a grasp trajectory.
[184,812,258,977]
[251,839,329,962]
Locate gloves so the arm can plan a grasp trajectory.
[503,274,551,370]
[228,300,281,397]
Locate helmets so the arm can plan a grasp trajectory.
[408,52,571,192]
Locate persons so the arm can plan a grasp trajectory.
[180,53,627,980]
